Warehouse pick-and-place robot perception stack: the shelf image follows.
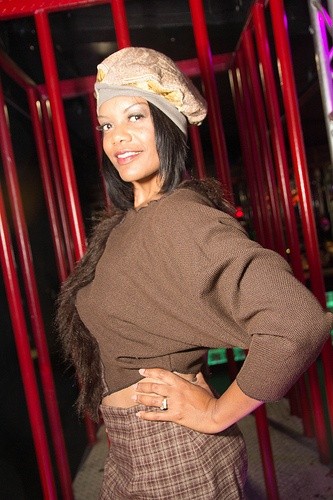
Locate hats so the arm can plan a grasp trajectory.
[93,48,207,141]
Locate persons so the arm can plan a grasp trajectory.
[237,178,333,312]
[57,46,330,499]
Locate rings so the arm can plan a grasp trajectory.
[159,396,168,411]
